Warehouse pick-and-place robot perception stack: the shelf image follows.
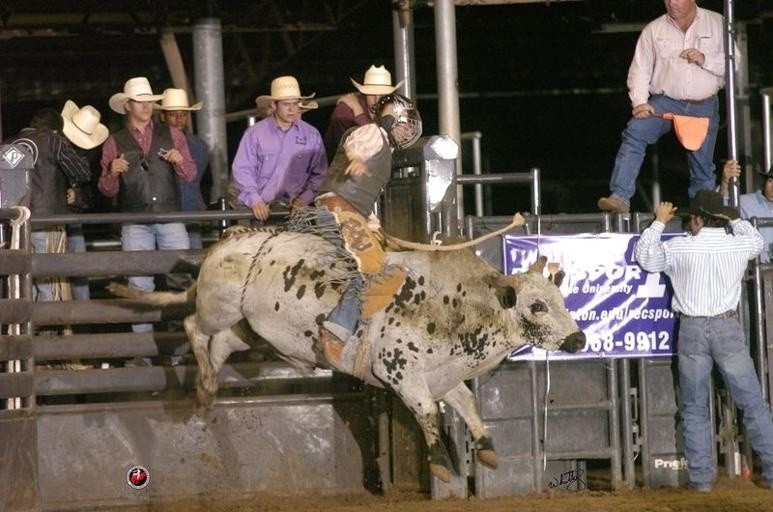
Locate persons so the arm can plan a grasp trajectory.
[635,189,773,492]
[312,93,424,368]
[153,87,212,249]
[324,64,405,158]
[97,76,198,367]
[715,159,773,264]
[231,75,329,225]
[598,0,742,213]
[61,99,110,370]
[4,107,92,370]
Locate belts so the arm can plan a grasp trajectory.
[661,94,719,104]
[677,311,736,320]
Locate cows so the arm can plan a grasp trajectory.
[108,224,587,485]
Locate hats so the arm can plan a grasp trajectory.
[754,164,772,180]
[58,96,111,150]
[254,74,316,107]
[672,188,740,222]
[153,89,205,113]
[108,78,162,115]
[296,99,318,110]
[350,63,405,96]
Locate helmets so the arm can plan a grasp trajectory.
[369,95,423,152]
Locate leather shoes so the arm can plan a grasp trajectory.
[124,357,150,368]
[315,331,346,371]
[597,195,630,215]
